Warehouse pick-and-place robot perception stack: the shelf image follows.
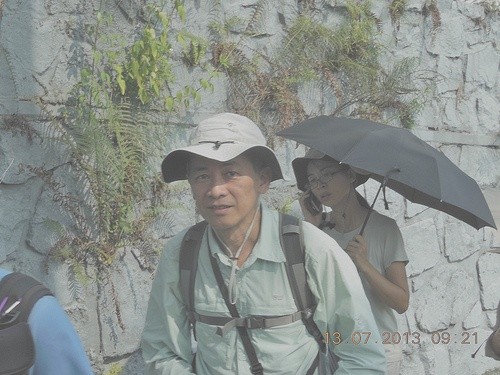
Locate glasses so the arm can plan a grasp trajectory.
[302,167,346,190]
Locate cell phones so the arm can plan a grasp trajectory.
[304,190,322,217]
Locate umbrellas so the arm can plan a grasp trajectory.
[272,115,498,244]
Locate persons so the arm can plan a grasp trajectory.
[139,112,387,374]
[291,147,411,375]
[0,268,97,375]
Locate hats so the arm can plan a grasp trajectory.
[156,113,285,183]
[291,148,373,196]
